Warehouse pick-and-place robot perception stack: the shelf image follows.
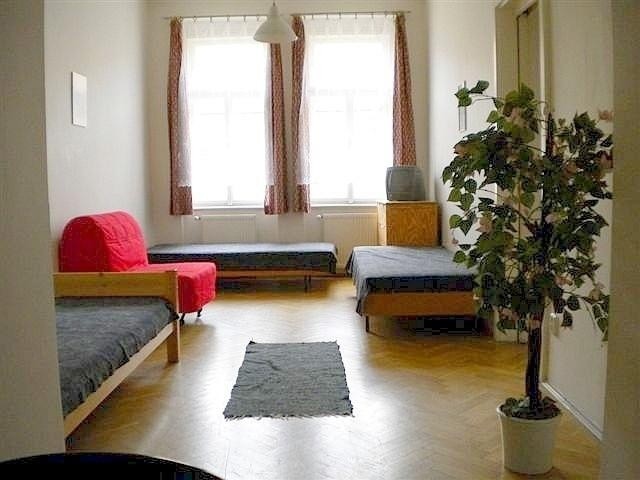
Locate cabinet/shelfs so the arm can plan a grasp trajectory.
[376,201,439,247]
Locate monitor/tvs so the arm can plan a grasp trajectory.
[386,165,426,200]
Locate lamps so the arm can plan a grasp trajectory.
[251,0,301,46]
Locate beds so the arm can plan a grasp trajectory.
[52,268,183,439]
[342,245,496,333]
[146,240,339,294]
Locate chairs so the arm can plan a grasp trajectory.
[56,210,217,327]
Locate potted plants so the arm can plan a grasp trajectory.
[440,79,610,475]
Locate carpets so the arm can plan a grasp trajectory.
[220,335,355,420]
